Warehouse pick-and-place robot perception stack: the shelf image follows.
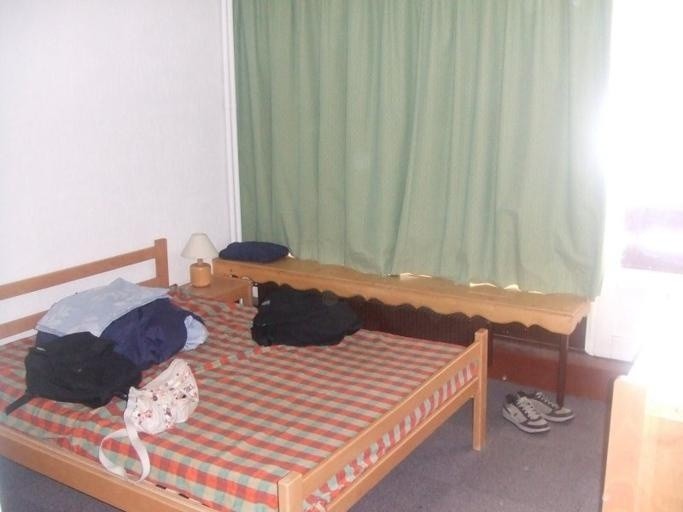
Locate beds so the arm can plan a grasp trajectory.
[0,234,491,512]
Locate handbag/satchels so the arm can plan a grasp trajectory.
[24,330,142,407]
[125,357,200,436]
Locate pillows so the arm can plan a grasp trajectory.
[219,241,289,263]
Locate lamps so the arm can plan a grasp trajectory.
[181,232,219,289]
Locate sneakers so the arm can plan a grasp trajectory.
[517,390,575,422]
[501,395,551,434]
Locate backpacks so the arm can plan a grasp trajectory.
[253,298,361,347]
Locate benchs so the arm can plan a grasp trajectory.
[210,253,591,408]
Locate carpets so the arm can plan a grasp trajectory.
[0,377,610,512]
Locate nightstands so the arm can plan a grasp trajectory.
[179,275,253,308]
